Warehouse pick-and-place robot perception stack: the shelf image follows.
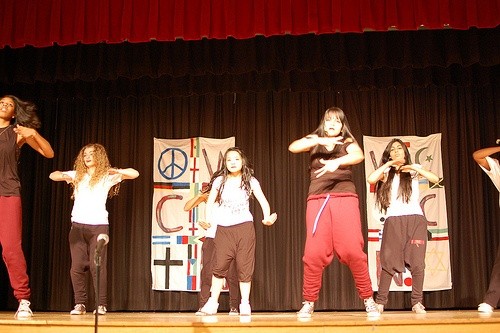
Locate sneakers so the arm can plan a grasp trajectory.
[363,296,381,317]
[17,299,32,317]
[93,303,107,314]
[70,302,86,314]
[296,301,315,318]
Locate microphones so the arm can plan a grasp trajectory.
[95,233,109,254]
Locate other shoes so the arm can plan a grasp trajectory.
[201,297,219,314]
[477,302,495,312]
[412,302,426,313]
[375,302,384,313]
[239,302,252,316]
[195,308,207,316]
[229,309,238,315]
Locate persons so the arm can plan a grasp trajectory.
[48,143,140,315]
[182,169,242,316]
[367,137,440,315]
[288,107,382,319]
[1,95,53,317]
[200,148,278,315]
[473,137,500,312]
